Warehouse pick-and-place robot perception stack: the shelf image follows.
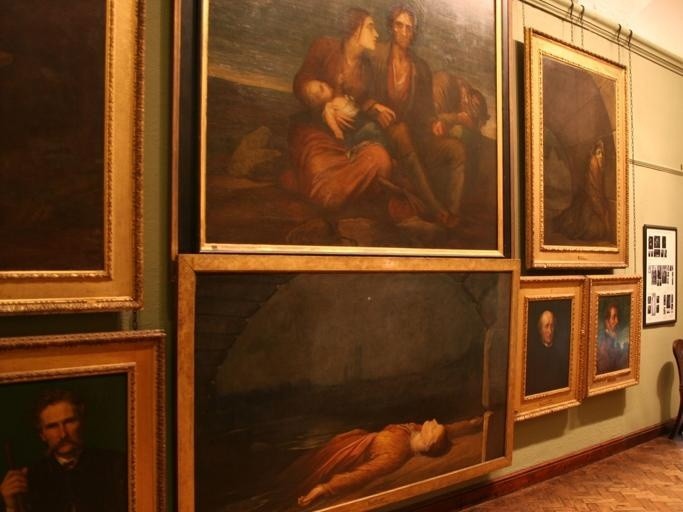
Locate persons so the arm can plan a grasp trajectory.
[525,310,569,396]
[562,141,614,242]
[2,387,126,512]
[297,417,480,509]
[596,300,629,375]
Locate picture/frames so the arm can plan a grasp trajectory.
[582,275,642,400]
[175,254,521,511]
[0,3,145,312]
[514,277,585,422]
[644,222,678,327]
[172,3,518,258]
[0,330,168,511]
[523,28,629,269]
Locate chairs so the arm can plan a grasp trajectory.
[668,339,683,439]
